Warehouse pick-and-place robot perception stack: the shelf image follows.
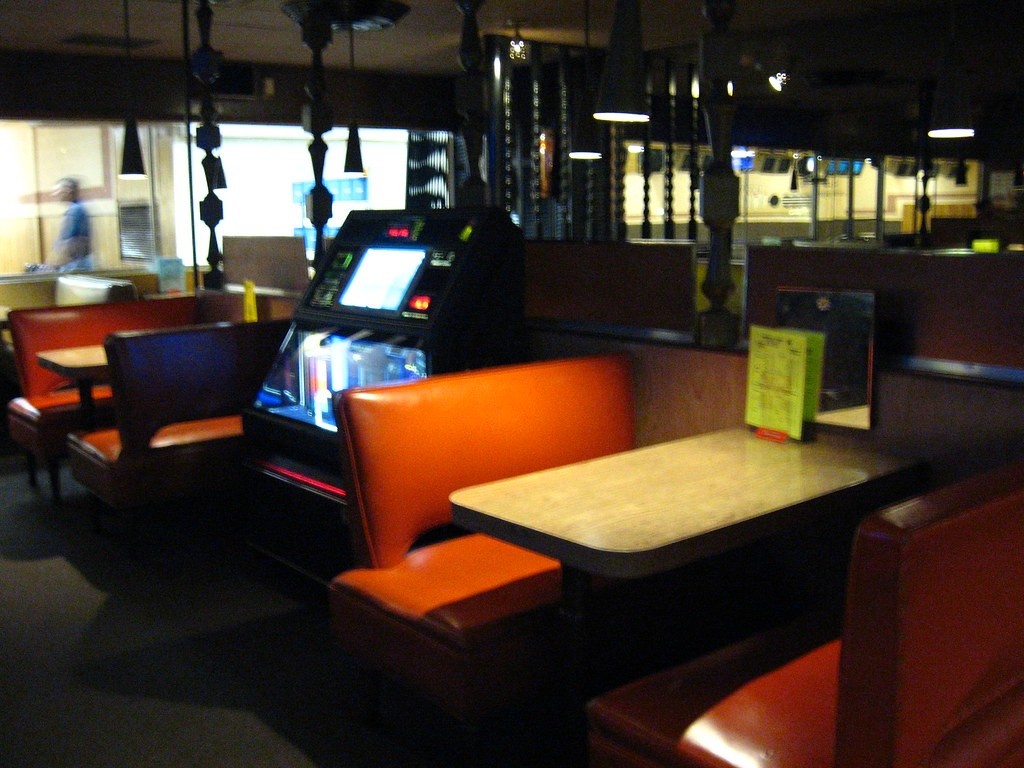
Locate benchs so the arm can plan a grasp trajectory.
[587,457,1024,768]
[67,315,293,553]
[6,293,199,487]
[329,352,715,768]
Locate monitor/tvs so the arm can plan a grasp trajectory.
[334,242,433,320]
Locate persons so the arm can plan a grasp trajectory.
[46,178,93,271]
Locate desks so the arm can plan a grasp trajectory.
[447,427,924,714]
[33,343,114,432]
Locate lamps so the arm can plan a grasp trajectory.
[767,65,793,93]
[504,18,530,62]
